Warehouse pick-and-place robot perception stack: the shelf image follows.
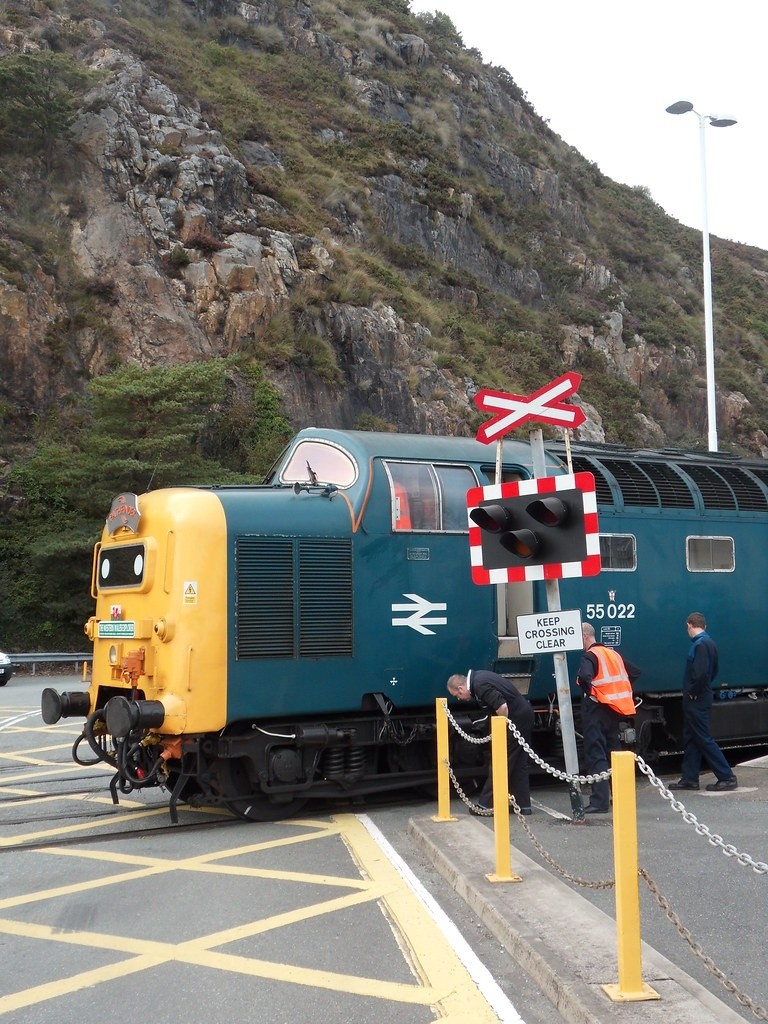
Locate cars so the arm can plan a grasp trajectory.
[1,652,12,686]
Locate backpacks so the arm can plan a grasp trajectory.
[460,733,487,769]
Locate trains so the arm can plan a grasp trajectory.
[41,424,768,821]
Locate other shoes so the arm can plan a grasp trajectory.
[509,805,532,815]
[469,805,494,817]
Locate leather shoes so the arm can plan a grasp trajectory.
[706,776,737,791]
[667,778,701,790]
[584,805,608,813]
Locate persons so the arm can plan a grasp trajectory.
[446,669,535,817]
[668,612,738,792]
[575,622,640,814]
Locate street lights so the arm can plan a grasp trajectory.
[666,100,738,455]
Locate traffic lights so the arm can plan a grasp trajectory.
[461,474,601,584]
